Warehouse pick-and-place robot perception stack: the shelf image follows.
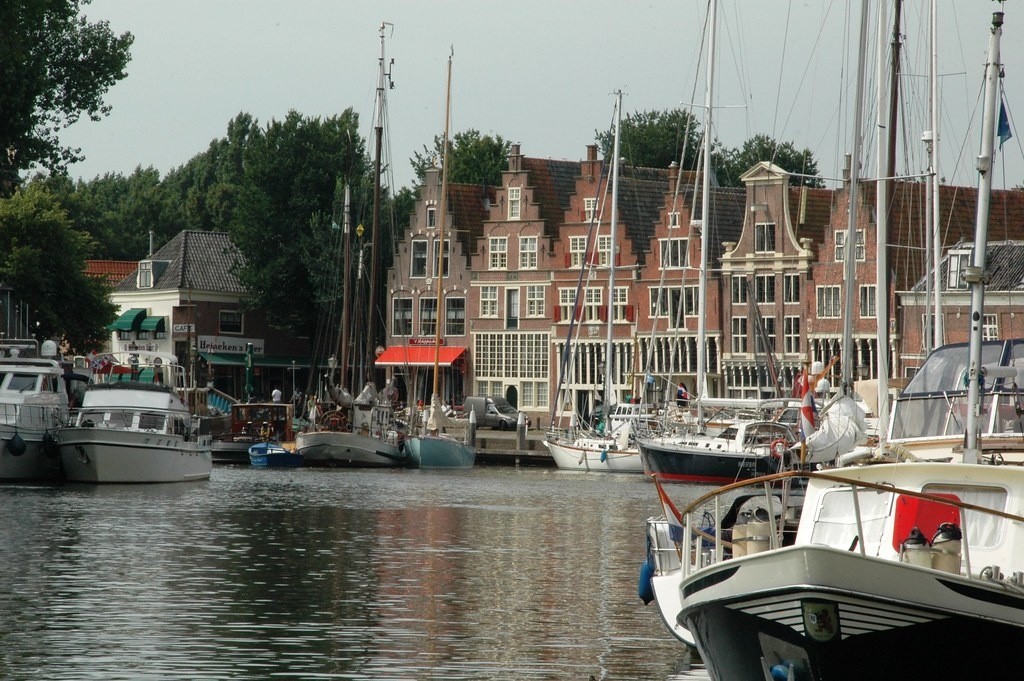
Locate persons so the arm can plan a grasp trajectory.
[441,400,452,417]
[272,384,282,420]
[289,388,314,419]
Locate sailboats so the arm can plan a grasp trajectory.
[272,0,1024,681]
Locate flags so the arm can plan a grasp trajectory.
[997,98,1012,151]
[84,350,119,371]
[595,387,602,402]
[677,382,687,399]
[646,373,656,384]
[799,367,818,443]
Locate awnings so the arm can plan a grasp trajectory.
[374,345,469,367]
[198,351,374,369]
[106,308,166,333]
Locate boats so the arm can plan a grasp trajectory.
[46,350,212,484]
[0,356,75,483]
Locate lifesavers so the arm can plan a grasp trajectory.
[769,439,790,460]
